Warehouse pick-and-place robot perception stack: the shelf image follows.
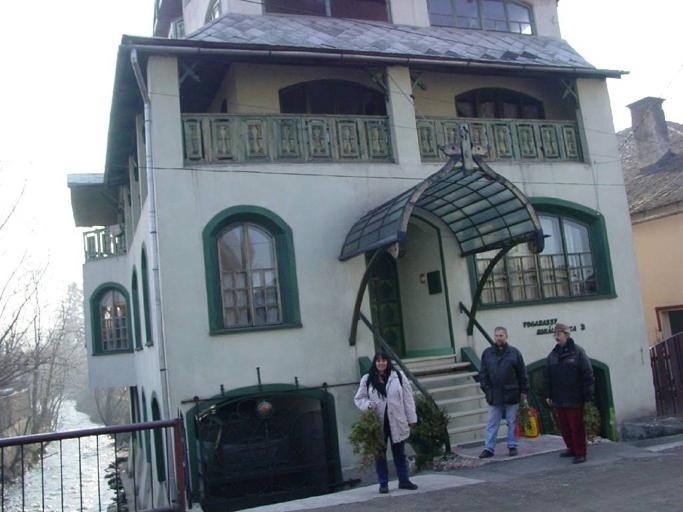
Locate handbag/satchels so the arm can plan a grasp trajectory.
[515,398,540,437]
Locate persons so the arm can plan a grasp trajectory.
[353,351,419,493]
[478,326,530,459]
[540,322,596,465]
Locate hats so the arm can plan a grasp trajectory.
[553,323,573,335]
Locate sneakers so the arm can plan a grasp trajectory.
[398,481,418,491]
[379,483,390,494]
[479,449,495,459]
[508,447,518,456]
[572,456,587,464]
[560,449,573,458]
